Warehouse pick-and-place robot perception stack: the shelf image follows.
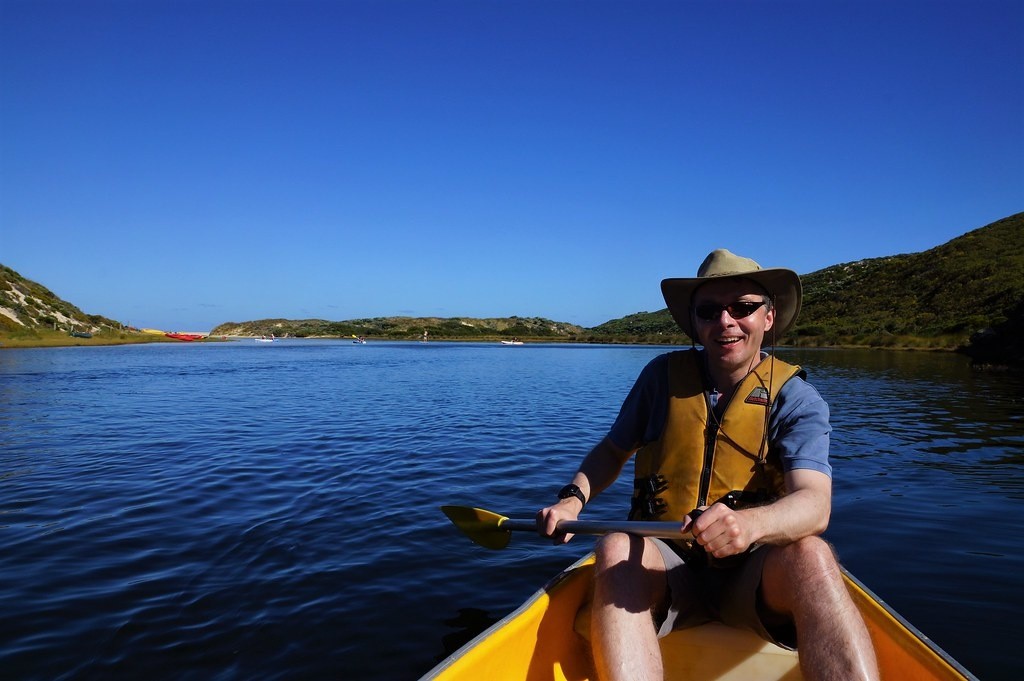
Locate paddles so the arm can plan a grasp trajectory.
[440,503,695,551]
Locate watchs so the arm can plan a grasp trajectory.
[557,484,585,509]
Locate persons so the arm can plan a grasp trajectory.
[539,249,879,681]
[261,334,265,339]
[270,334,274,340]
[358,336,364,342]
[513,336,518,342]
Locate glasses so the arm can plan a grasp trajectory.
[692,302,768,320]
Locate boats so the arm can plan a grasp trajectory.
[254,338,279,342]
[419,534,980,681]
[501,340,523,345]
[353,340,366,344]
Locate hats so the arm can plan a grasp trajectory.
[660,249,802,348]
